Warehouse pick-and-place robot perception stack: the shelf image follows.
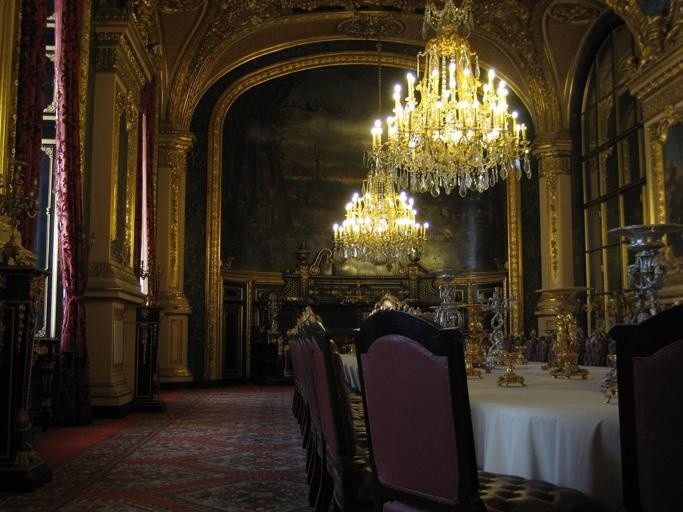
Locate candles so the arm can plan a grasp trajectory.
[424,228,425,236]
[522,127,526,140]
[334,230,338,238]
[388,123,391,136]
[372,136,376,148]
[410,83,414,99]
[420,226,422,234]
[513,119,516,133]
[378,133,381,144]
[516,131,519,145]
[340,232,342,237]
[354,202,356,209]
[489,79,494,97]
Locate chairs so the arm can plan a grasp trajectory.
[355,308,480,512]
[301,321,361,512]
[609,300,683,512]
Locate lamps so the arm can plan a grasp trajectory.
[332,12,427,265]
[362,1,532,197]
